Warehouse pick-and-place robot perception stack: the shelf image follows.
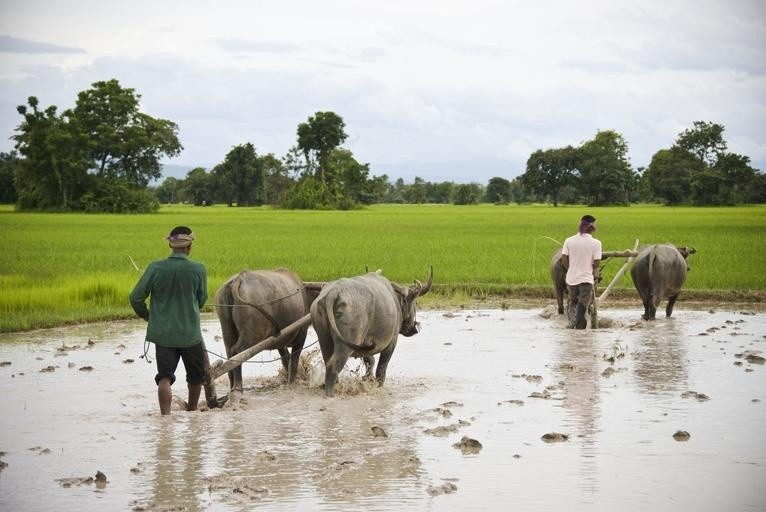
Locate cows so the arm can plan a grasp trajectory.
[550,249,606,316]
[308,263,436,400]
[213,265,323,403]
[629,240,699,322]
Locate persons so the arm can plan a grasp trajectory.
[559,213,603,331]
[127,224,208,416]
